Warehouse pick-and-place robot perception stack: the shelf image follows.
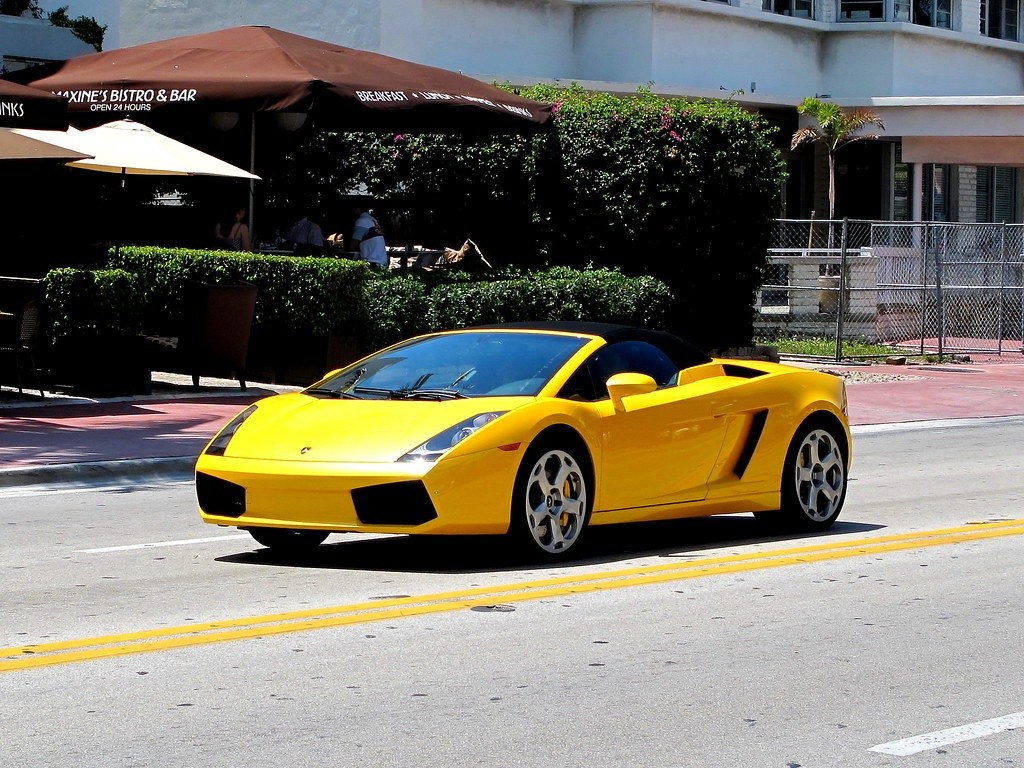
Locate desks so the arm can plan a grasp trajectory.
[386,250,443,270]
[251,249,295,255]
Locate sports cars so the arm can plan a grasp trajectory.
[194,326,853,559]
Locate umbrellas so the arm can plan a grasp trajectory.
[0,111,260,249]
[33,22,557,247]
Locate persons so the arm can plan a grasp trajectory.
[282,204,325,248]
[345,200,389,271]
[213,206,253,252]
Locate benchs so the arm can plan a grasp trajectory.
[392,262,477,286]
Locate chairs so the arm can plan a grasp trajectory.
[589,340,679,394]
[476,343,530,387]
[0,299,52,398]
